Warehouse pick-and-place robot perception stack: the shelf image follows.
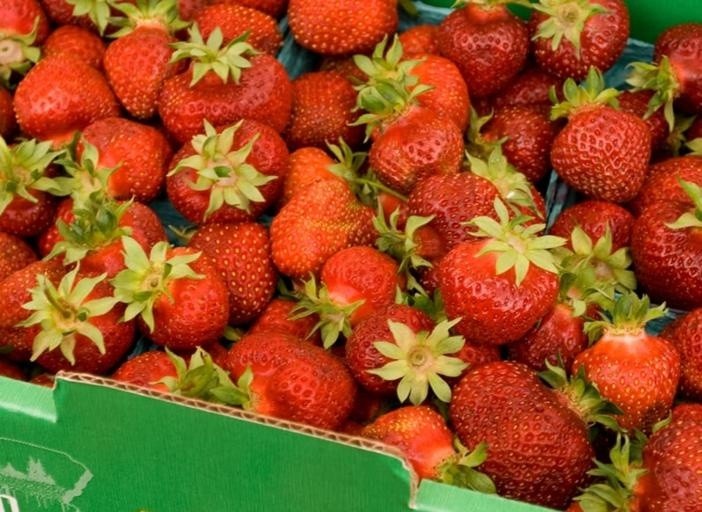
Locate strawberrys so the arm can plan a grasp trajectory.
[0,1,702,512]
